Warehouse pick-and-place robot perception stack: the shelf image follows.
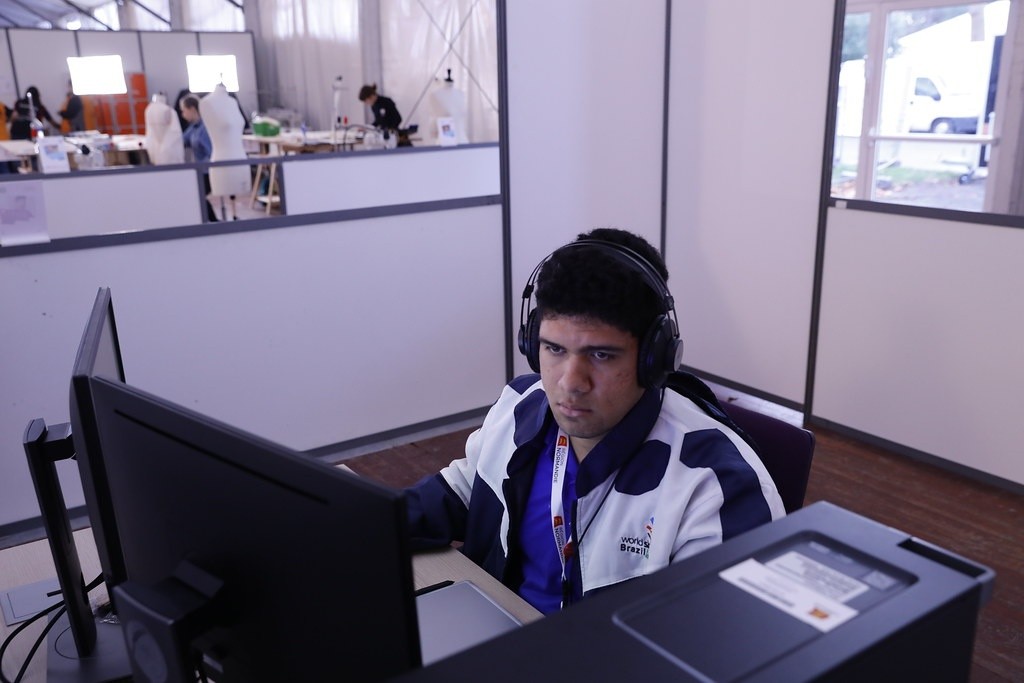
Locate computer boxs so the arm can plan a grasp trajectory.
[402,500,996,682]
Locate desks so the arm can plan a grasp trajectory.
[1,462,549,682]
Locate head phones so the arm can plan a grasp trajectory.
[518,240,684,389]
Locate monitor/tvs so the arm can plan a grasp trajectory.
[70,285,424,683]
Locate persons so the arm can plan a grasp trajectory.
[396,229,788,617]
[144,94,184,165]
[198,82,252,195]
[359,82,402,139]
[178,93,219,223]
[431,79,465,145]
[7,80,86,140]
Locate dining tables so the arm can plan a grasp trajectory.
[0,122,393,220]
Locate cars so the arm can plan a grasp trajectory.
[909,76,983,137]
